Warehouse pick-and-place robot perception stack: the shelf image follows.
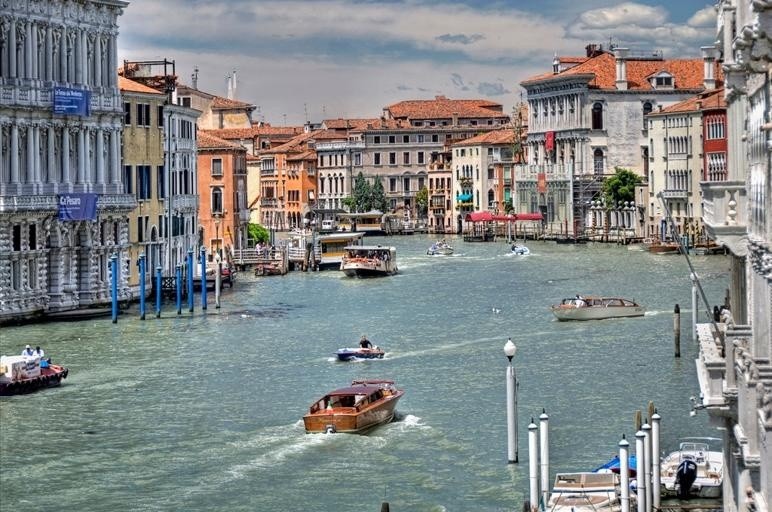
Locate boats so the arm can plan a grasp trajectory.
[315,207,414,277]
[543,433,726,512]
[304,377,408,435]
[510,246,530,255]
[428,244,454,255]
[332,344,386,364]
[0,354,70,396]
[551,292,646,322]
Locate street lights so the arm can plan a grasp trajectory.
[500,336,522,462]
[215,215,220,255]
[686,271,701,341]
[213,254,222,311]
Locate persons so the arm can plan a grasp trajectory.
[21,344,35,357]
[574,295,587,311]
[712,303,735,325]
[32,345,45,359]
[253,237,450,265]
[673,454,699,498]
[511,244,519,252]
[358,335,373,350]
